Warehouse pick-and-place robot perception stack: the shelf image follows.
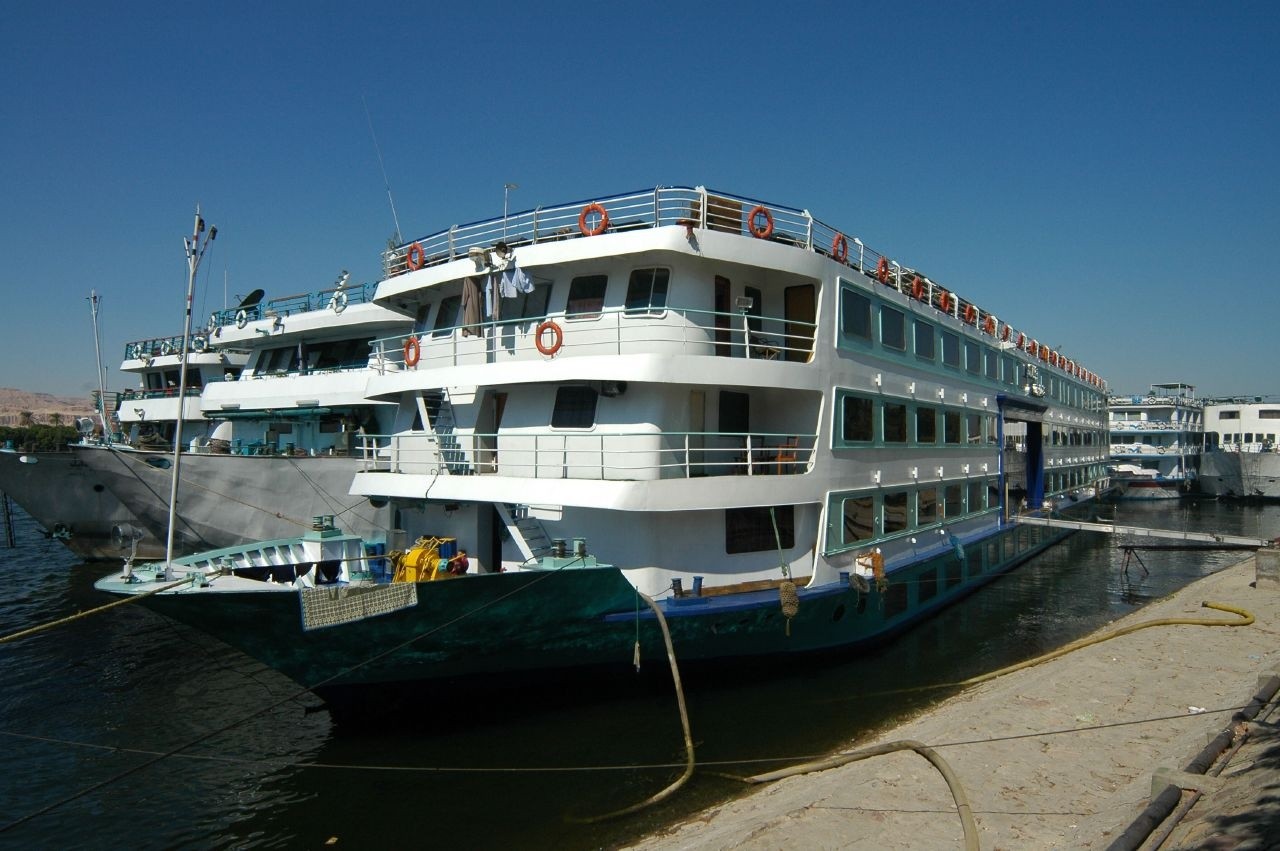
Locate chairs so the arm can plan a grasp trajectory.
[749,333,781,361]
[743,436,763,474]
[774,436,799,476]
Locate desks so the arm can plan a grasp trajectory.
[733,456,776,475]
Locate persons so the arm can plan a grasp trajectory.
[450,549,469,577]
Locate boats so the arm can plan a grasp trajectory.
[1197,394,1280,498]
[66,281,389,556]
[88,184,1111,721]
[1107,383,1204,498]
[0,333,226,541]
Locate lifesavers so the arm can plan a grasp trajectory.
[235,310,247,329]
[579,203,608,236]
[965,305,974,324]
[832,234,848,263]
[877,256,889,282]
[912,276,924,299]
[746,205,773,238]
[207,315,215,333]
[534,321,563,354]
[332,292,348,314]
[982,316,1106,389]
[191,336,208,354]
[132,345,142,359]
[160,341,170,355]
[406,244,424,270]
[940,290,950,313]
[403,339,420,368]
